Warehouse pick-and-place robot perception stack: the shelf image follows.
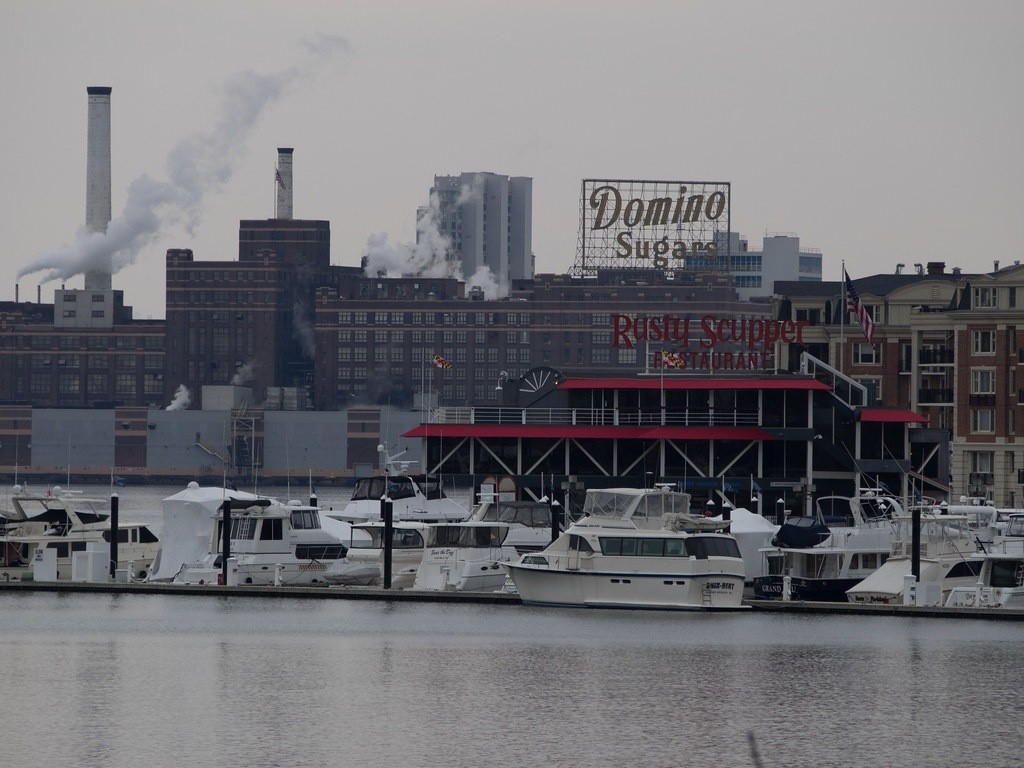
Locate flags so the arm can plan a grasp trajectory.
[844,268,876,353]
[663,350,686,370]
[431,353,452,368]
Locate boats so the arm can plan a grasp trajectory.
[0,467,1024,619]
